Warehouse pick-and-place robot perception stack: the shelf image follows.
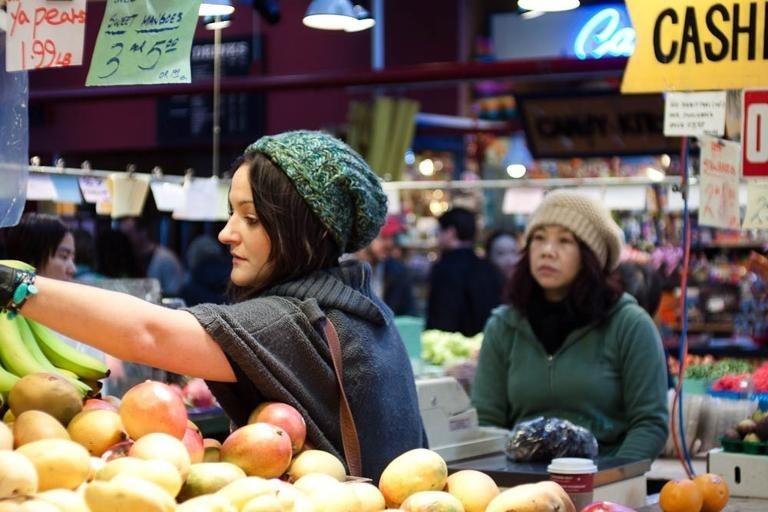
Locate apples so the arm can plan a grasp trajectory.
[580,501,634,512]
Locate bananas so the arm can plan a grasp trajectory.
[0,259,111,399]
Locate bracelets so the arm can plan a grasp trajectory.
[8,268,36,323]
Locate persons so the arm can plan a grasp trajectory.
[1,206,232,308]
[472,187,669,463]
[345,205,683,342]
[0,126,429,491]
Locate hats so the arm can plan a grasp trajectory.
[521,187,626,276]
[378,214,408,239]
[241,126,390,257]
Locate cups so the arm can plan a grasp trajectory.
[547,456,597,512]
[393,316,427,365]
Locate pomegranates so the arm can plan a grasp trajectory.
[1,372,347,479]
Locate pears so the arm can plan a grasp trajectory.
[1,432,574,512]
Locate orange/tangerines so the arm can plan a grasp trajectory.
[659,479,704,512]
[693,473,729,512]
